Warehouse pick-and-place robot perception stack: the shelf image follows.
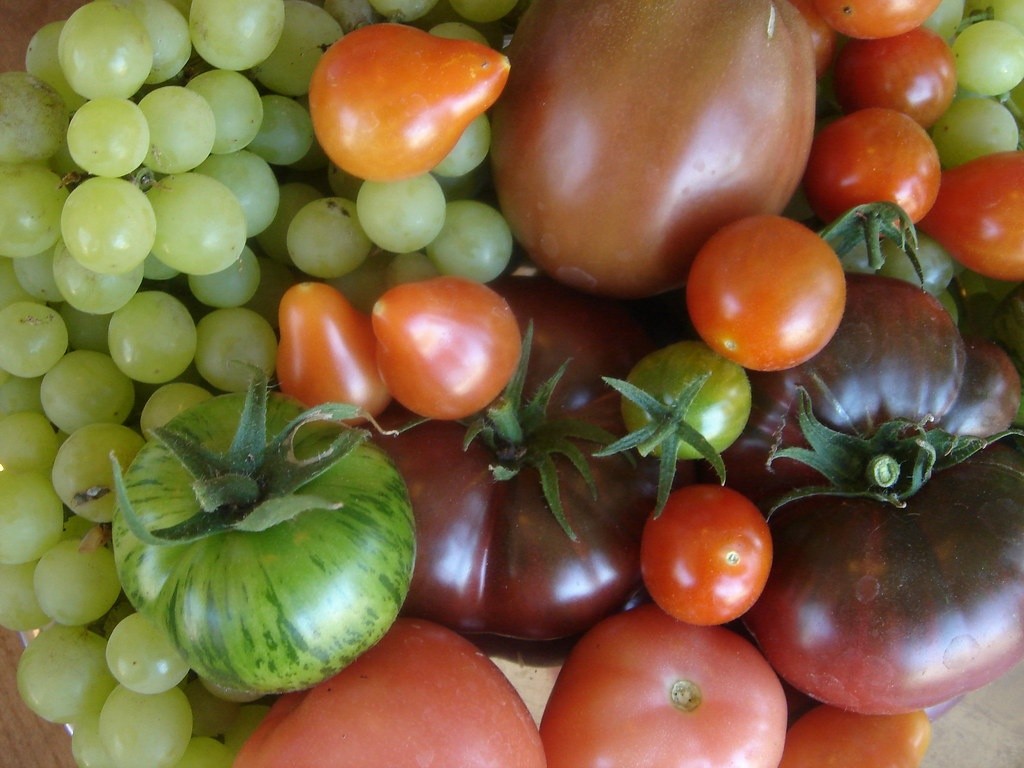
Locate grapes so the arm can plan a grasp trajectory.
[0,0,1024,768]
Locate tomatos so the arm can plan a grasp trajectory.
[114,0,1024,767]
